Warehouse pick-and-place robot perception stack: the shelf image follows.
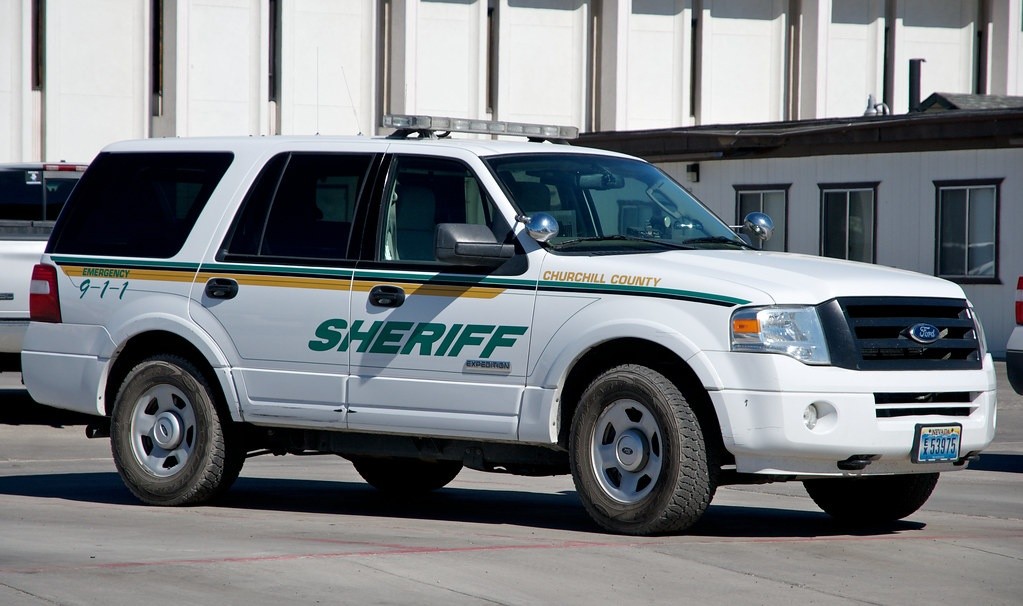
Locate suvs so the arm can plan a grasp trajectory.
[0,162,92,354]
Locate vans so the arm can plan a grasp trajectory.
[20,114,999,537]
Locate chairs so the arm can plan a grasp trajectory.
[492,185,554,263]
[380,186,452,269]
